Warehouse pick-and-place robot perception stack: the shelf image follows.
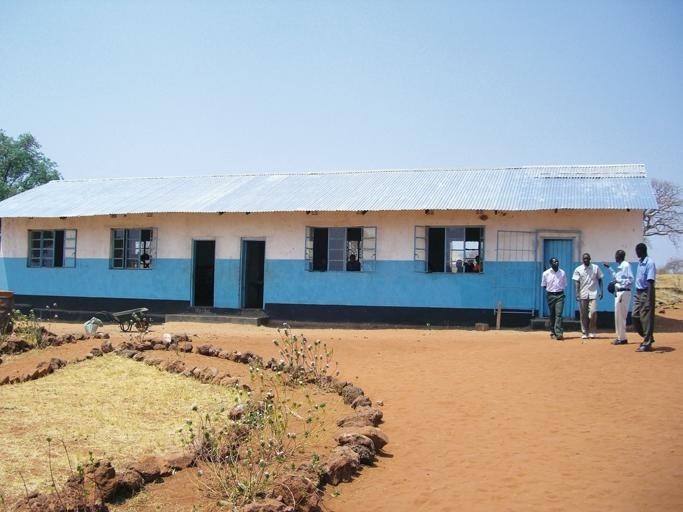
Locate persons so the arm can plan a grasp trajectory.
[604,249,634,345]
[541,258,568,339]
[572,253,604,339]
[346,254,361,271]
[455,255,480,273]
[631,242,657,352]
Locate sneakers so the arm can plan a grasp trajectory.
[556,331,627,344]
[635,342,651,352]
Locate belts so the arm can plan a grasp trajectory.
[635,287,648,290]
[617,287,631,290]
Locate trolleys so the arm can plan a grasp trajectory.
[92,307,151,333]
